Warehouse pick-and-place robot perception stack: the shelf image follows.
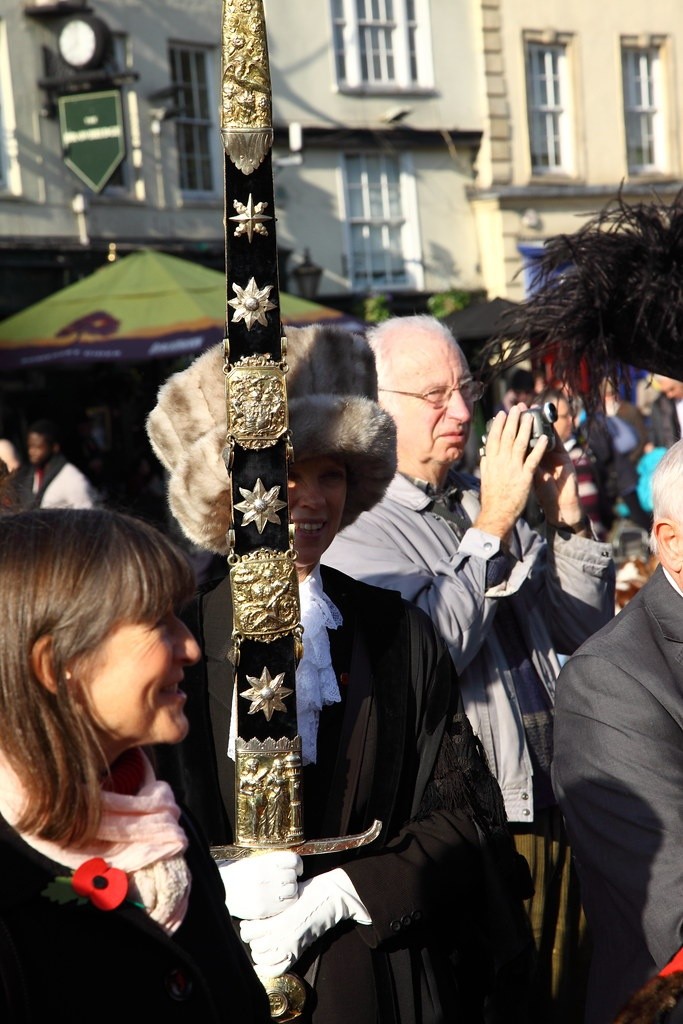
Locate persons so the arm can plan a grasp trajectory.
[147,325,556,1024]
[455,328,683,611]
[0,507,275,1024]
[0,395,169,531]
[554,434,683,1024]
[318,315,615,1024]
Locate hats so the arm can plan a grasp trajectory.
[144,323,397,557]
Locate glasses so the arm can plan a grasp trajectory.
[378,380,488,408]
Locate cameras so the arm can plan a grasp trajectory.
[476,401,559,457]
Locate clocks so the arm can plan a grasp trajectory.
[54,13,104,71]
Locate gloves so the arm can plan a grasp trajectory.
[239,868,373,979]
[218,851,304,920]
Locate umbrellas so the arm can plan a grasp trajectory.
[0,243,362,374]
